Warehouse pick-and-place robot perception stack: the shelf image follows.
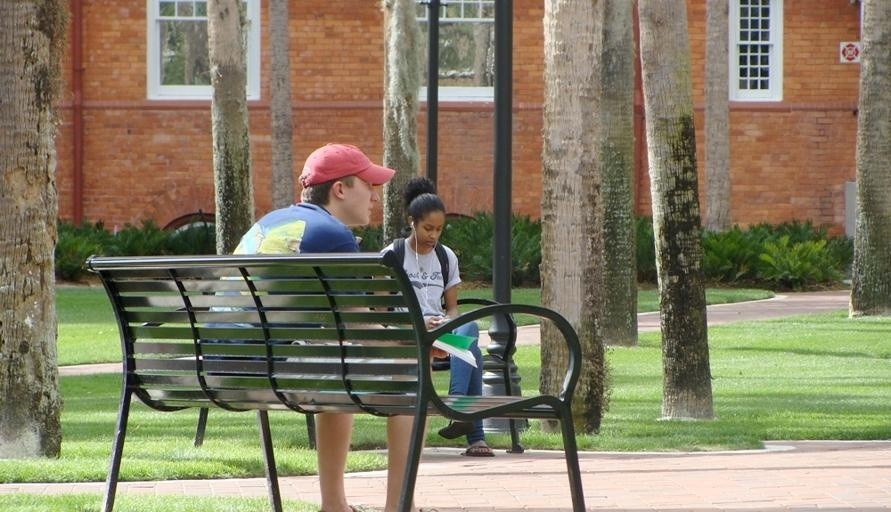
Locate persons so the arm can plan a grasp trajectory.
[371,176,496,458]
[198,142,441,512]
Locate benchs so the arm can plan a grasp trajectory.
[83,251,585,512]
[194,298,525,456]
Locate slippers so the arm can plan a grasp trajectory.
[460,446,495,457]
[437,421,474,439]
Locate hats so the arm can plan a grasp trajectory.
[301,142,396,186]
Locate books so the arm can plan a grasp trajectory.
[432,333,478,369]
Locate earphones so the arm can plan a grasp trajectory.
[411,221,414,226]
